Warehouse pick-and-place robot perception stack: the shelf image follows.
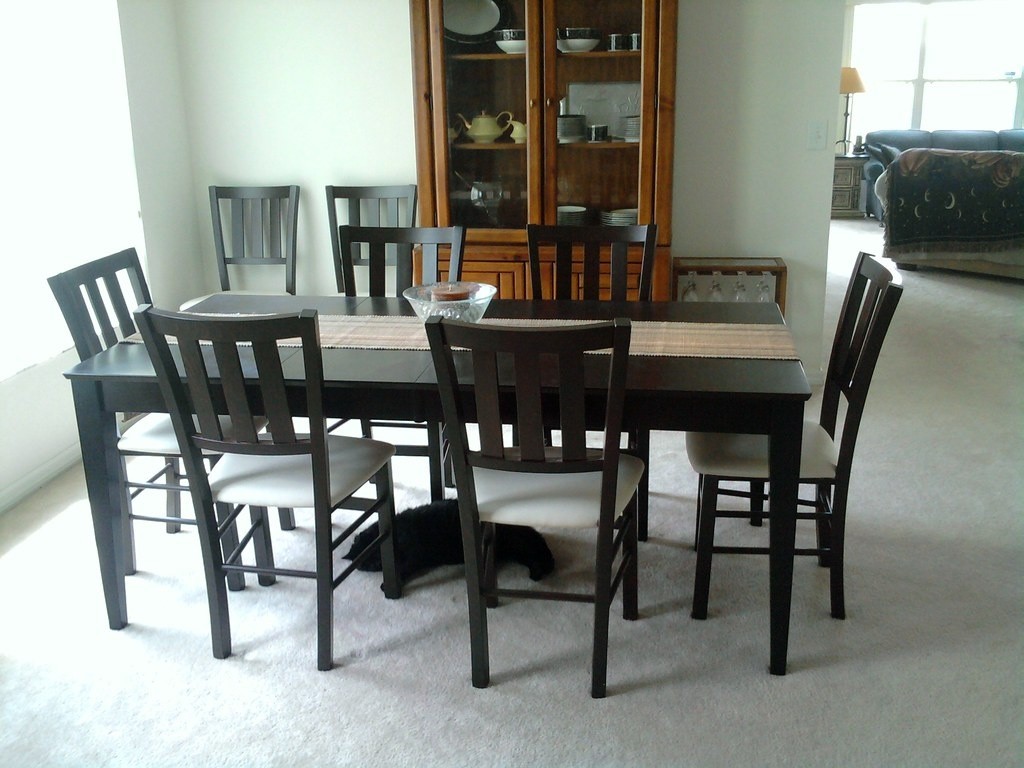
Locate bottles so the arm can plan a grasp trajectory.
[682,272,774,303]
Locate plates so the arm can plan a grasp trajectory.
[557,115,587,143]
[624,115,640,142]
[442,0,513,53]
[599,208,637,227]
[558,206,587,226]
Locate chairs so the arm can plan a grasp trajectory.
[525,224,658,542]
[50,247,294,591]
[339,224,466,499]
[426,315,644,699]
[208,185,300,296]
[327,185,416,291]
[688,251,906,622]
[134,306,397,669]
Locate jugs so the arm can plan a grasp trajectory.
[506,120,527,145]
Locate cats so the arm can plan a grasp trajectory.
[341,497,554,593]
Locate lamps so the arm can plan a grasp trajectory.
[837,67,864,154]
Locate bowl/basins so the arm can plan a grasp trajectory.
[401,281,498,326]
[556,27,603,53]
[493,28,526,55]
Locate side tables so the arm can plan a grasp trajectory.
[831,154,870,218]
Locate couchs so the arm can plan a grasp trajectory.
[859,128,1024,280]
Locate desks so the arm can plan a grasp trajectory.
[67,291,811,675]
[672,258,788,315]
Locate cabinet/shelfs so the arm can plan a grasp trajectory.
[409,0,678,303]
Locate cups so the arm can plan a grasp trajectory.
[608,33,640,51]
[588,125,608,143]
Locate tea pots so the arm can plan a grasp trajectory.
[457,110,514,144]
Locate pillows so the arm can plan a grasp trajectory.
[867,143,889,174]
[877,141,901,159]
